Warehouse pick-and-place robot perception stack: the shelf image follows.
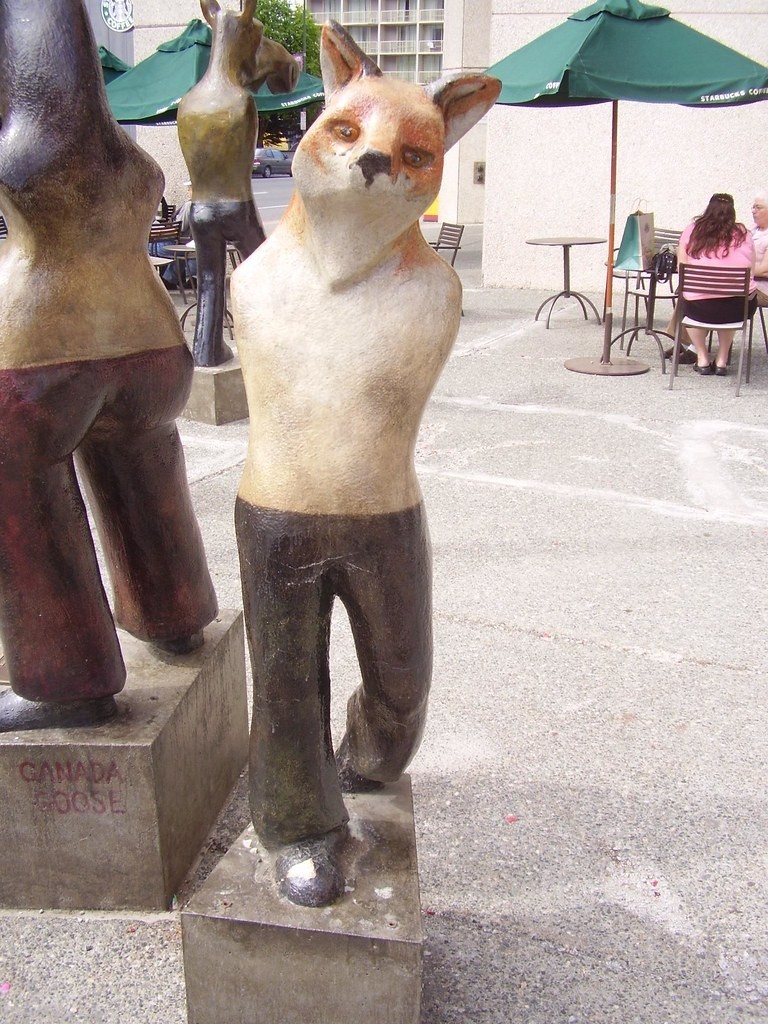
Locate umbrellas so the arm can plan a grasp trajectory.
[105,18,324,127]
[98,46,132,86]
[483,0,768,367]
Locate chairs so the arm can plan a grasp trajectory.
[603,227,768,397]
[0,195,241,304]
[428,222,464,317]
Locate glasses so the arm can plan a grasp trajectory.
[752,207,766,211]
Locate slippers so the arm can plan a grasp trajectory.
[712,359,727,375]
[693,361,712,374]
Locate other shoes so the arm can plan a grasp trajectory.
[664,343,686,358]
[669,348,698,363]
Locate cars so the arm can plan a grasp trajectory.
[253,148,292,178]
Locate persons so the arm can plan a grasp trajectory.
[663,191,768,364]
[677,193,755,376]
[149,181,197,291]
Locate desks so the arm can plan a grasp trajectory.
[162,244,243,340]
[524,236,607,330]
[601,259,685,374]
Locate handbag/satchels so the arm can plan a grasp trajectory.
[651,249,677,284]
[614,198,655,271]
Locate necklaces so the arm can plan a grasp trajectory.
[756,226,761,232]
